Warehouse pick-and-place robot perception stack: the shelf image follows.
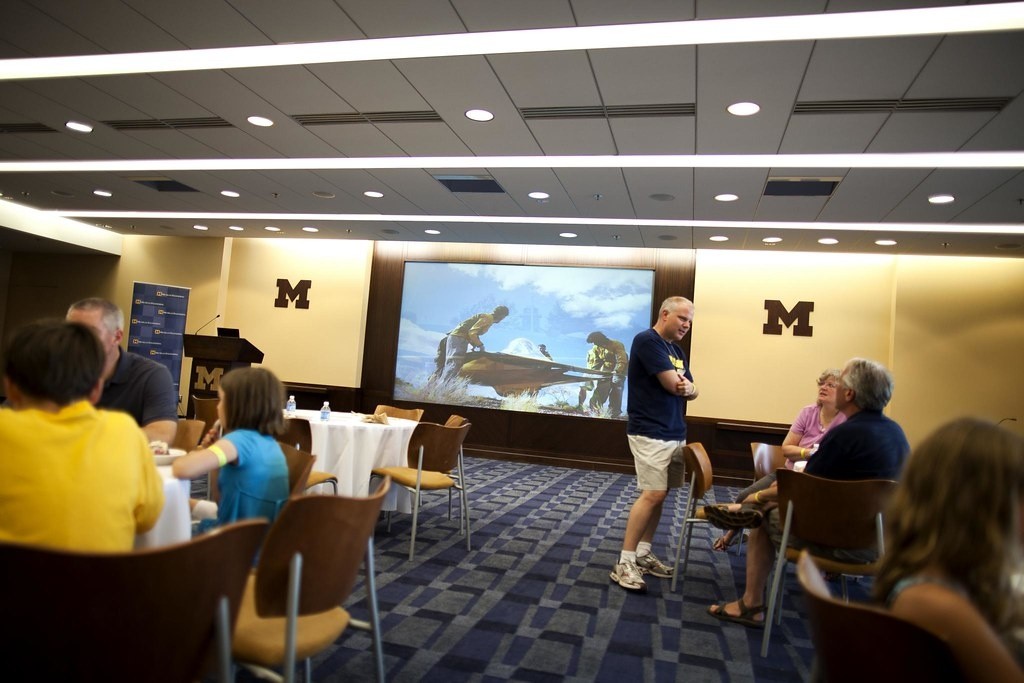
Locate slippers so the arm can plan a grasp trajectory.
[714,534,748,551]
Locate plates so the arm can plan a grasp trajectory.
[152,449,187,465]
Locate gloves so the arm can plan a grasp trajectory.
[612,376,620,384]
[478,343,484,351]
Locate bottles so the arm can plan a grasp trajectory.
[285,396,296,418]
[320,402,331,422]
[810,443,819,456]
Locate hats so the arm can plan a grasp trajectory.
[494,305,509,316]
[587,332,601,342]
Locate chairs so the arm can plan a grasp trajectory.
[670,441,971,683]
[0,402,473,683]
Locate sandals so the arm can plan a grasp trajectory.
[703,503,763,528]
[707,599,766,628]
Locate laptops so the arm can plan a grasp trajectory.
[217,327,239,337]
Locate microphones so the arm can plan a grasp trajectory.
[997,418,1017,425]
[195,315,221,335]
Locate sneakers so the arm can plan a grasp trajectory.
[636,550,675,578]
[610,560,648,591]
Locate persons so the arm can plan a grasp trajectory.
[66,298,178,447]
[527,344,553,405]
[427,324,454,394]
[579,332,629,417]
[0,319,162,555]
[713,369,848,552]
[437,306,509,401]
[172,368,290,565]
[703,357,912,627]
[609,296,697,590]
[874,417,1024,683]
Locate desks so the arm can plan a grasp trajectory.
[134,447,192,550]
[284,408,424,530]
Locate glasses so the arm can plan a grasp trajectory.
[835,375,850,390]
[818,381,837,389]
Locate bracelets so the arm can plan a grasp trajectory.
[755,491,762,504]
[801,448,805,459]
[692,383,696,395]
